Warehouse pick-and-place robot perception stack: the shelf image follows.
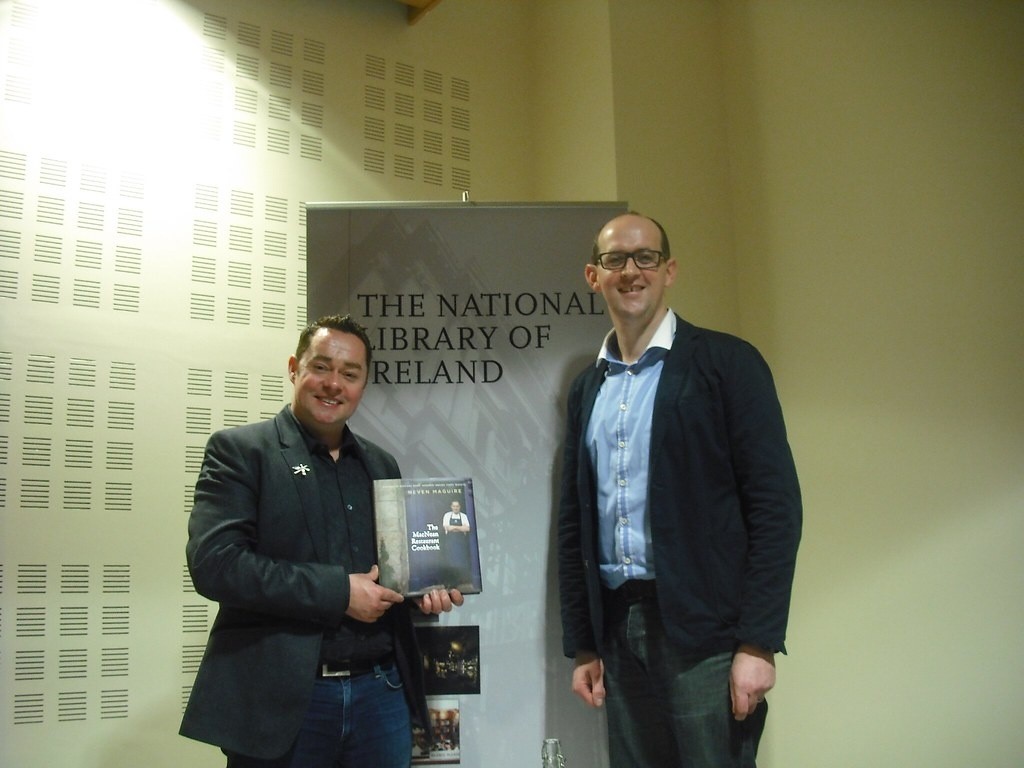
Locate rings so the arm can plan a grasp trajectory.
[758,698,766,705]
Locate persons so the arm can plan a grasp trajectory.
[442,498,470,587]
[554,209,804,768]
[177,314,464,768]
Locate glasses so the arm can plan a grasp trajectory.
[594,250,666,270]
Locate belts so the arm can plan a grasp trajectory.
[315,651,395,679]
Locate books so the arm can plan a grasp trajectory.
[372,476,482,598]
